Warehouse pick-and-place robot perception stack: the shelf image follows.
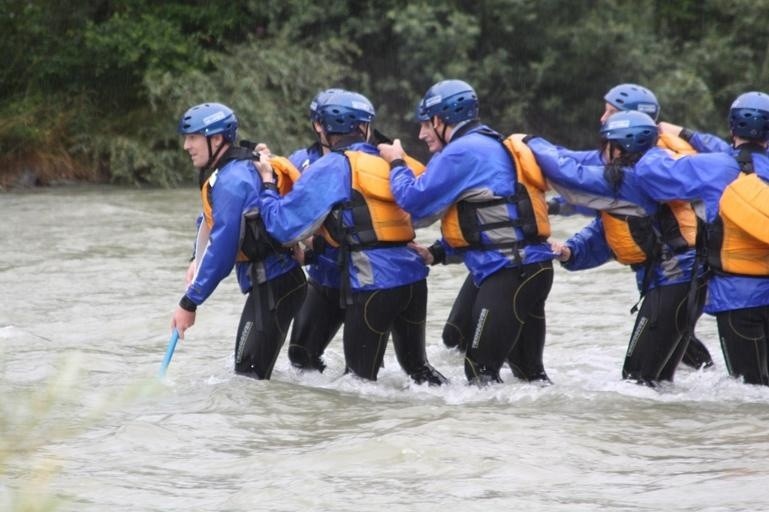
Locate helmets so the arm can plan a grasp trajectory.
[730,92,769,140]
[177,102,237,142]
[416,79,479,121]
[599,83,660,153]
[310,88,375,133]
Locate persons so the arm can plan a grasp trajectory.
[171,102,309,381]
[253,80,769,388]
